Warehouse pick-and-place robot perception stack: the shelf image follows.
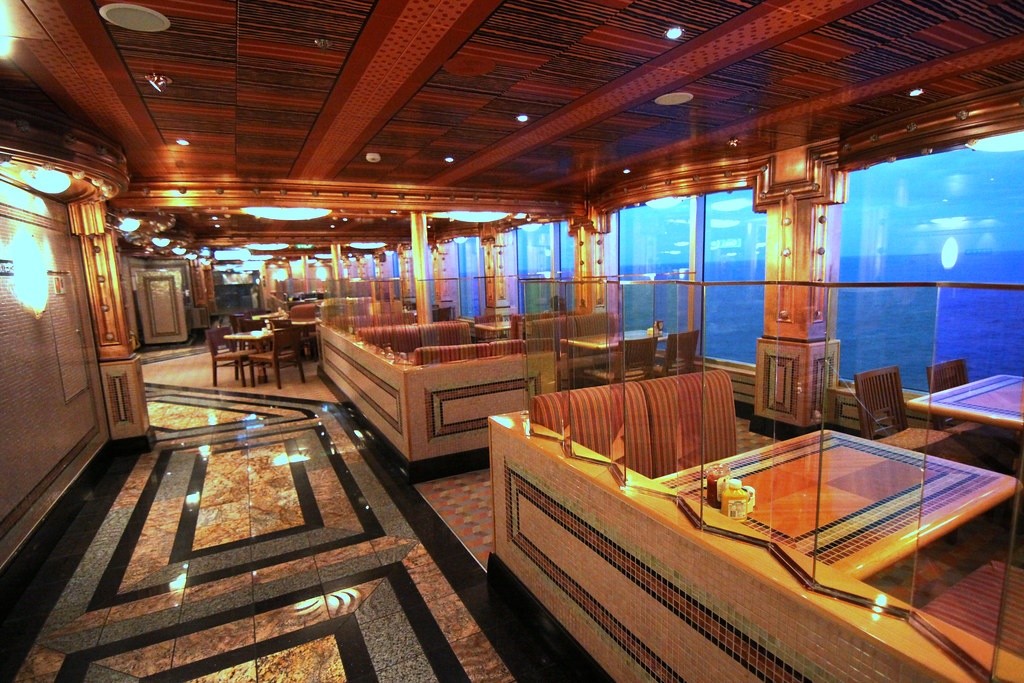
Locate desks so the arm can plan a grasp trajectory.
[651,429,1023,580]
[559,330,669,386]
[224,330,274,384]
[474,321,511,332]
[906,374,1024,430]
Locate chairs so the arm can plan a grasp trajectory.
[205,314,317,389]
[475,313,510,342]
[578,330,700,388]
[854,359,1015,546]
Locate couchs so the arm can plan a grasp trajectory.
[317,312,555,484]
[511,310,608,339]
[488,370,1024,683]
